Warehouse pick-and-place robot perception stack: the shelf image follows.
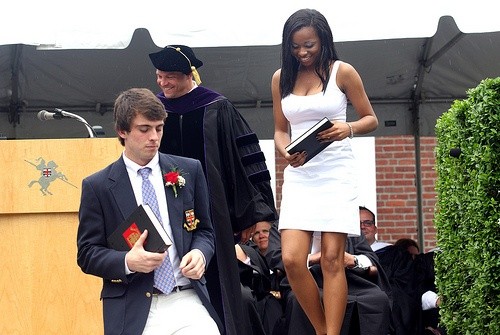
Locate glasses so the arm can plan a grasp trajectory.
[360,219,374,227]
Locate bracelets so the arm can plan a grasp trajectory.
[346,121,353,139]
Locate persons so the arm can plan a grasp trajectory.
[233,234,271,301]
[411,250,449,335]
[145,45,278,335]
[360,205,412,335]
[76,87,226,335]
[239,220,273,264]
[271,9,379,335]
[395,238,419,262]
[266,217,393,334]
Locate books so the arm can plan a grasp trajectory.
[284,117,335,166]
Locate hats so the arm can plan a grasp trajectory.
[138,168,176,295]
[148,44,203,74]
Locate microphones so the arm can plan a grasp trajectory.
[38,110,67,121]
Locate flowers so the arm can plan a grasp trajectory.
[161,166,186,199]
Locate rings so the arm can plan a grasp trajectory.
[251,231,255,237]
[327,136,332,140]
